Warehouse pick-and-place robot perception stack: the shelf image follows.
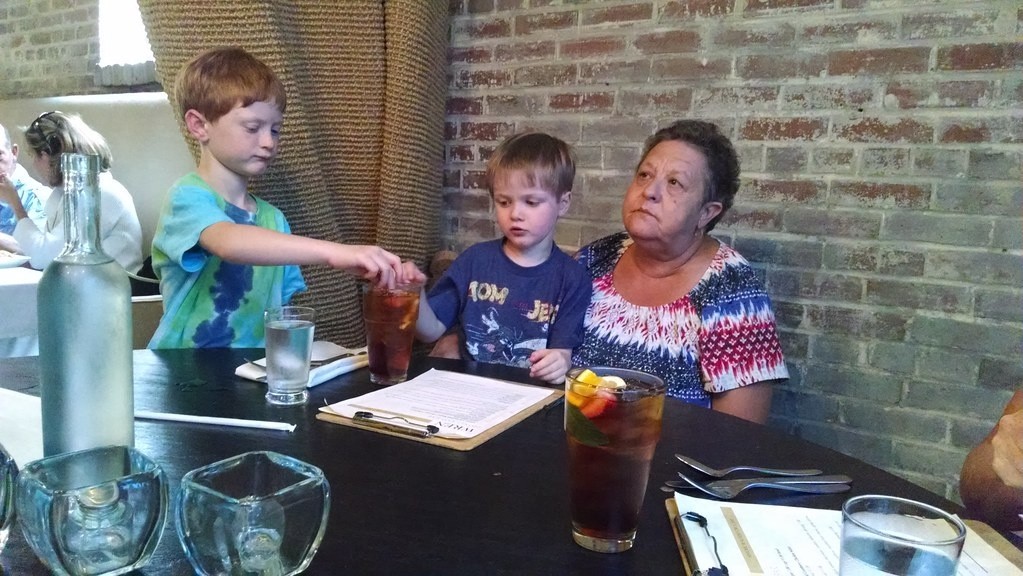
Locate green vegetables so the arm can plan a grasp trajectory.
[565,400,610,445]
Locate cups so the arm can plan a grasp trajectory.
[361,279,422,386]
[16,446,169,576]
[563,368,667,551]
[840,494,966,576]
[175,451,331,575]
[0,444,19,545]
[262,306,316,406]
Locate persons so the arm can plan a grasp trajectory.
[402,132,593,385]
[0,123,43,254]
[428,119,789,425]
[1,110,145,277]
[960,389,1023,530]
[148,45,401,349]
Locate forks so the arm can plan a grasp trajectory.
[244,357,266,371]
[677,472,850,499]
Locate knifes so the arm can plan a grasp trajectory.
[665,474,853,488]
[256,376,267,381]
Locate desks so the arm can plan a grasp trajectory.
[1,348,1023,576]
[0,268,42,337]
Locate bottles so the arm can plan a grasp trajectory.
[37,153,134,462]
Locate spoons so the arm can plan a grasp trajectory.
[675,454,823,477]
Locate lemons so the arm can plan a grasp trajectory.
[567,369,602,408]
[598,376,627,389]
